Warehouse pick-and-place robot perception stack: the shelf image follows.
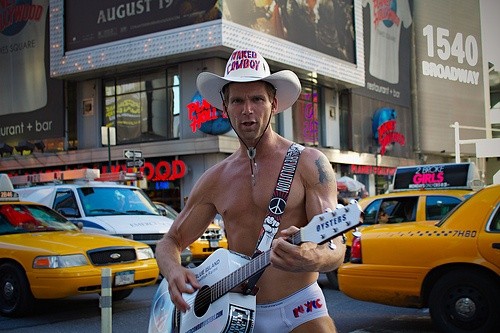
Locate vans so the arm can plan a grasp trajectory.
[0,186,193,273]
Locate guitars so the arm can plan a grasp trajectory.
[148,199,365,333]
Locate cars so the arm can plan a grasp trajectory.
[1,203,160,317]
[152,200,229,265]
[326,191,479,282]
[337,183,500,333]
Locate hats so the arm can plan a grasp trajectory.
[196,49,302,115]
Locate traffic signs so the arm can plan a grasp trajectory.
[122,148,145,187]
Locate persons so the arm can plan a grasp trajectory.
[153,48,347,333]
